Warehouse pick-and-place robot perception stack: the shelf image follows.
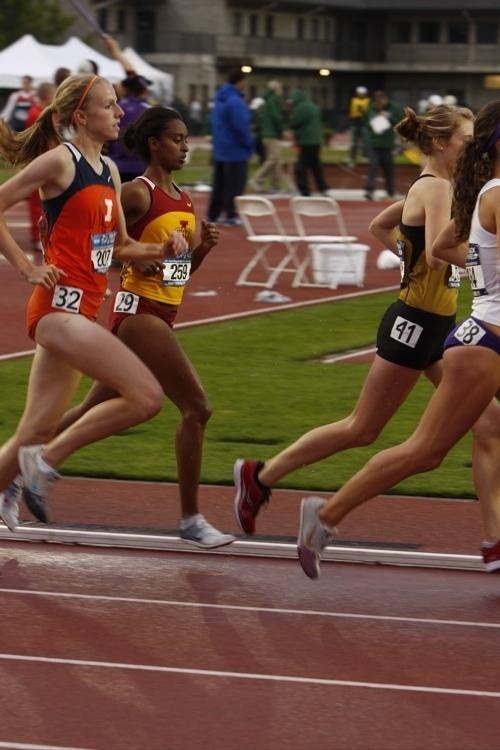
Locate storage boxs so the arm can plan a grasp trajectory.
[308,244,370,286]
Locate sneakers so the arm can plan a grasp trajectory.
[234,458,270,536]
[18,444,55,523]
[297,497,336,578]
[206,217,244,225]
[179,515,235,549]
[481,540,500,572]
[1,474,22,533]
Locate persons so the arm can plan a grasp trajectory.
[0,106,237,551]
[1,36,154,266]
[204,67,327,228]
[349,86,406,200]
[234,104,500,575]
[296,101,500,580]
[0,72,166,523]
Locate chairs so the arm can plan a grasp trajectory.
[290,196,364,291]
[232,196,310,289]
